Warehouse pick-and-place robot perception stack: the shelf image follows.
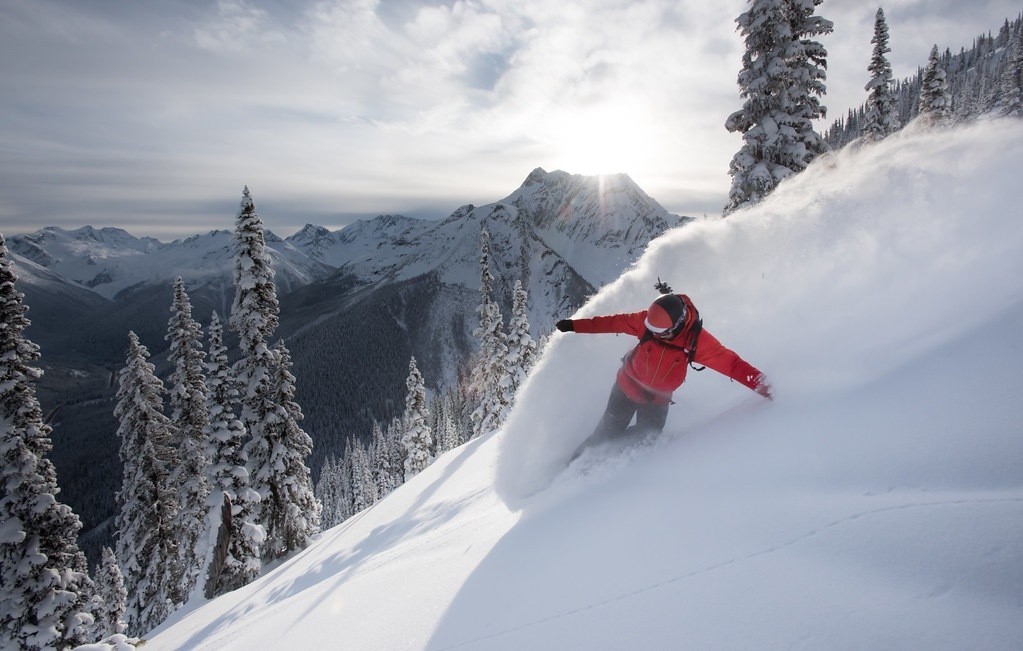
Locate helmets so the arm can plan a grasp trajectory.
[644,295,687,332]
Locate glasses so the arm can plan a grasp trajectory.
[652,331,673,338]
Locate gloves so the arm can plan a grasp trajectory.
[556,319,574,332]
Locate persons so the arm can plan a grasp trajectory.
[552,293,775,470]
